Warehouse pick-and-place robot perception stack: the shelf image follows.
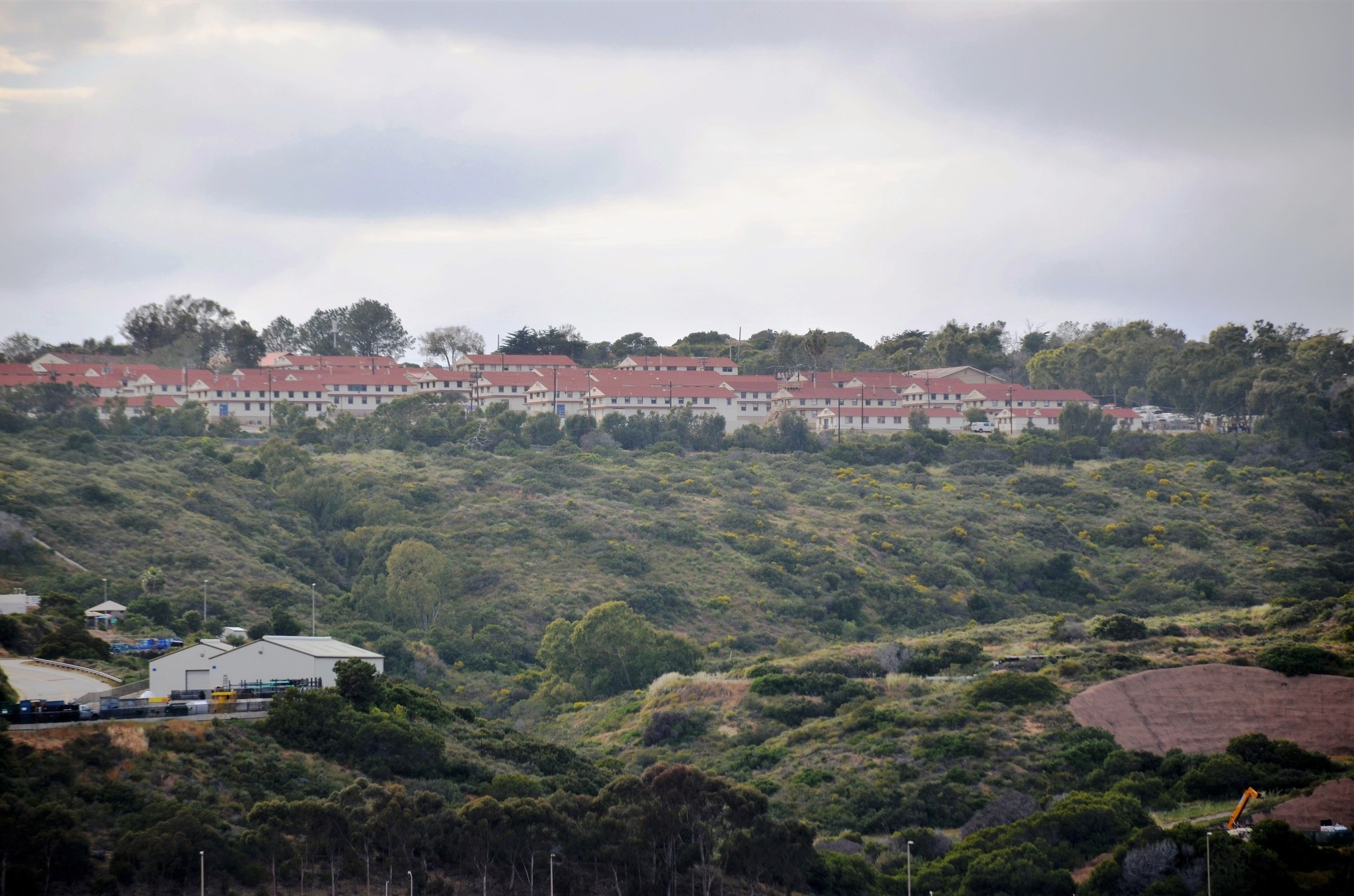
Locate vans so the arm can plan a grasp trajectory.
[971,422,996,433]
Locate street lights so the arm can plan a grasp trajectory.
[102,579,107,602]
[312,583,316,637]
[203,580,208,620]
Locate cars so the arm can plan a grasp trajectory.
[1132,405,1193,423]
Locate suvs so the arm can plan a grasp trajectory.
[57,702,101,722]
